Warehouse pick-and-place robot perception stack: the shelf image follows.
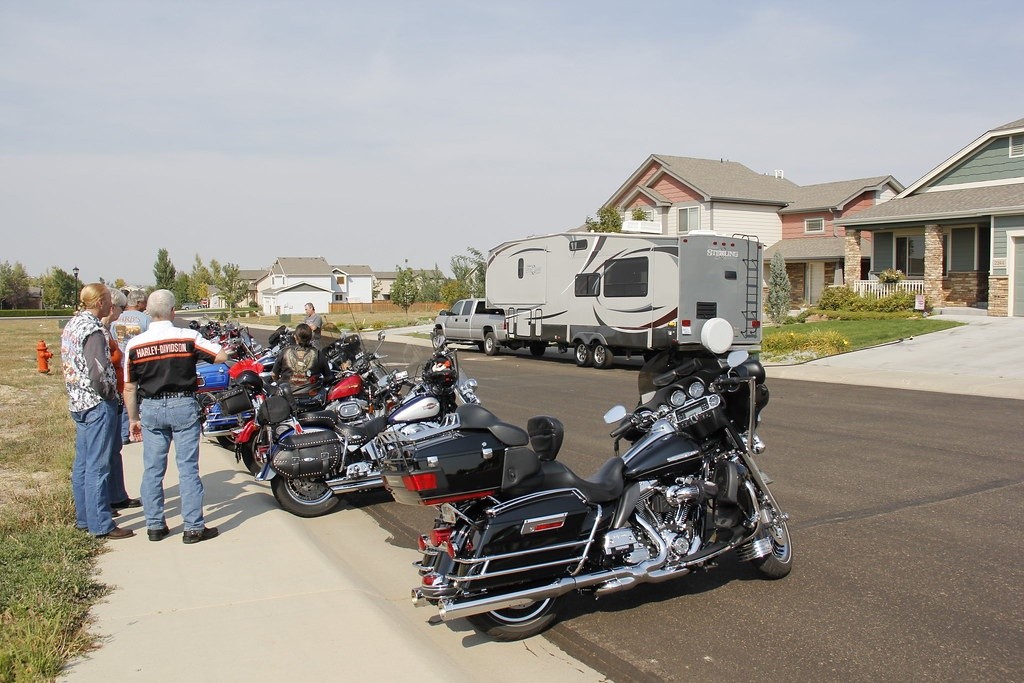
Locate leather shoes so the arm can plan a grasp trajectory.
[79,527,88,531]
[147,524,170,541]
[96,524,133,539]
[110,498,141,508]
[184,527,219,544]
[112,508,118,516]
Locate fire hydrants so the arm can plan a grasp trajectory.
[35,339,54,374]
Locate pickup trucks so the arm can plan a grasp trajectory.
[430,298,547,356]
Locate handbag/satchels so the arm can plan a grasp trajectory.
[220,385,253,415]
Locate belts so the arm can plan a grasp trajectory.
[145,391,194,400]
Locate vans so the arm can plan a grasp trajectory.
[181,303,202,310]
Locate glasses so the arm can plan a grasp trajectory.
[120,305,126,311]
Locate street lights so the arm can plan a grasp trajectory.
[72,265,79,311]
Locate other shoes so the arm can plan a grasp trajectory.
[122,439,131,444]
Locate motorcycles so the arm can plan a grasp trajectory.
[188,319,410,476]
[254,343,483,518]
[379,342,794,644]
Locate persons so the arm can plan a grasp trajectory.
[304,302,322,351]
[121,289,229,544]
[61,282,156,540]
[271,324,335,399]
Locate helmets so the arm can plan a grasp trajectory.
[189,321,198,329]
[421,352,459,388]
[234,369,263,393]
